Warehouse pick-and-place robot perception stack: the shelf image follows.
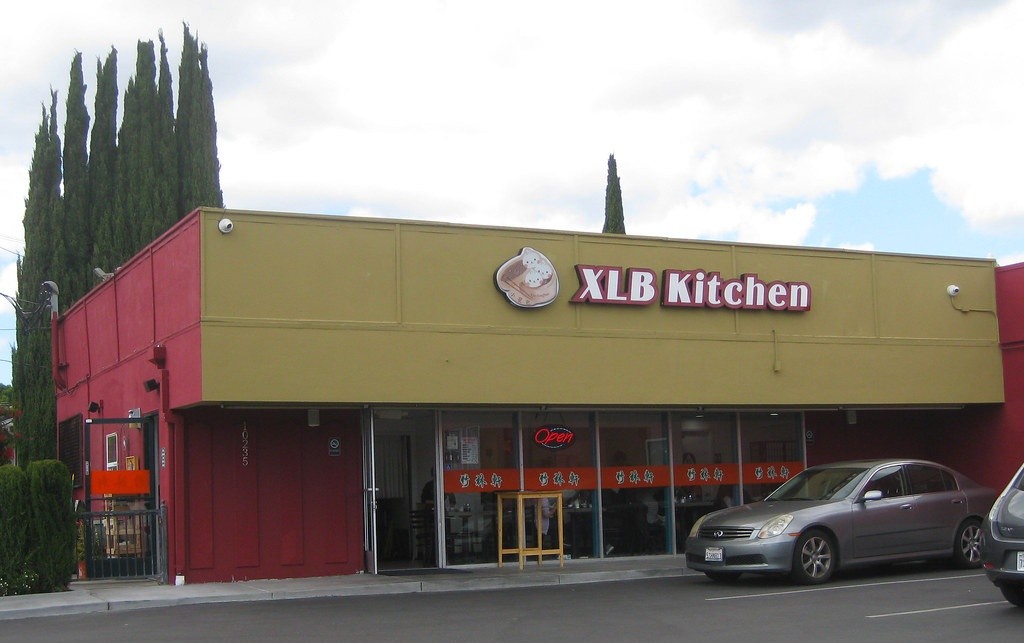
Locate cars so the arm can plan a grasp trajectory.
[450,510,497,554]
[979,461,1024,607]
[684,459,1002,585]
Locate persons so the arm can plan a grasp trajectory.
[420,466,456,568]
[480,451,721,565]
[715,484,733,511]
